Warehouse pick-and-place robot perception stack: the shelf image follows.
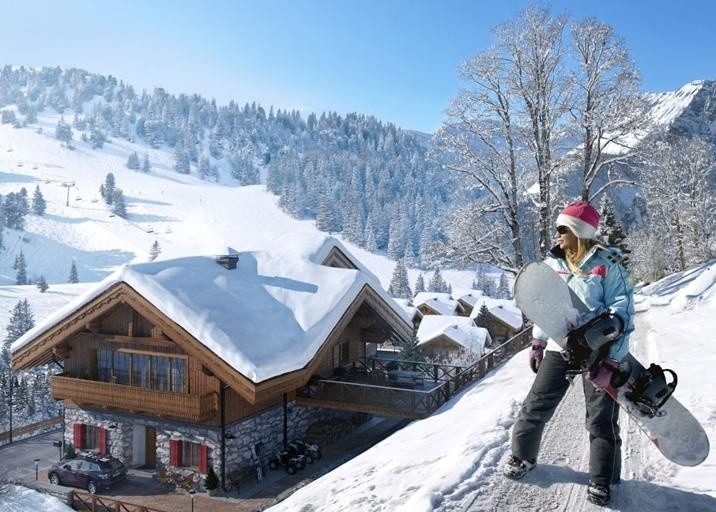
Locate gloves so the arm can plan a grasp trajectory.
[585,357,619,393]
[529,337,547,372]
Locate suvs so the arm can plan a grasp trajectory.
[287,438,321,465]
[268,446,306,476]
[46,452,127,495]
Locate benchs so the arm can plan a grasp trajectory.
[383,379,416,389]
[228,465,259,495]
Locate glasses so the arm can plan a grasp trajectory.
[556,224,568,234]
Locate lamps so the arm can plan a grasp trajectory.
[109,420,117,429]
[226,431,236,439]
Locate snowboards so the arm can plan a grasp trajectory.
[513,261,710,467]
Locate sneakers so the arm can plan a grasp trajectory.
[504,455,537,479]
[588,480,610,506]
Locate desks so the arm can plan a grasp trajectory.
[388,370,424,387]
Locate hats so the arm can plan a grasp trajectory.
[555,200,601,239]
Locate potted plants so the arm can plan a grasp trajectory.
[202,463,220,496]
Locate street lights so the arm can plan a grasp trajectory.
[3,366,20,444]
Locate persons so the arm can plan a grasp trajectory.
[503,201,635,506]
